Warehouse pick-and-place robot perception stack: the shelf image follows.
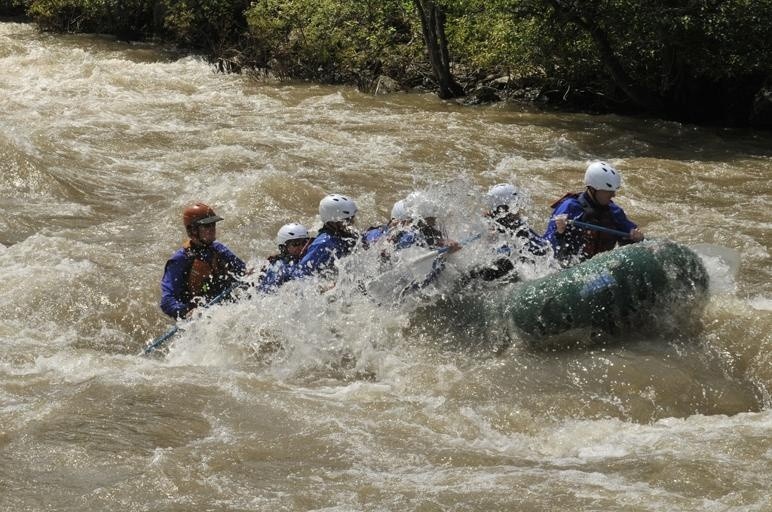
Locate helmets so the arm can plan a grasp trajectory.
[585,162,620,192]
[391,199,411,221]
[184,203,223,238]
[319,194,357,224]
[276,223,310,246]
[488,183,517,208]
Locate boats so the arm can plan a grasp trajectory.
[152,238,709,364]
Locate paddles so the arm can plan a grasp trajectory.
[566,217,740,278]
[400,233,480,278]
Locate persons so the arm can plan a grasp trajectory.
[257,223,309,293]
[160,202,254,320]
[362,191,459,285]
[461,181,547,284]
[291,191,359,280]
[543,161,644,270]
